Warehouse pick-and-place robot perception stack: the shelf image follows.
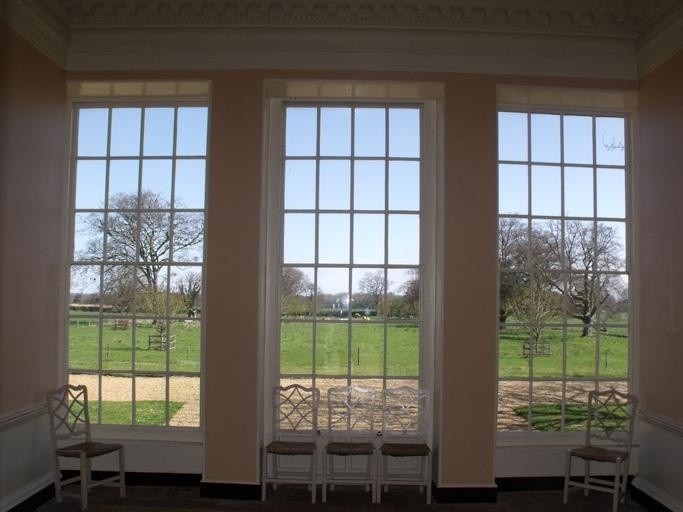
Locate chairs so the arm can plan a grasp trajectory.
[46,385,125,511]
[562,391,638,511]
[262,384,431,505]
